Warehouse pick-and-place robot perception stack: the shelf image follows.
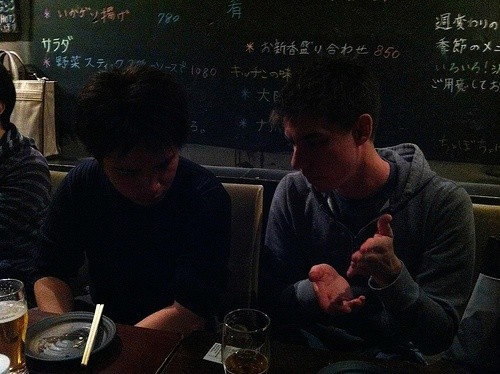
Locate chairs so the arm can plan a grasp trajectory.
[220,182,267,317]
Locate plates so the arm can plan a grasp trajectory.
[25,311,117,362]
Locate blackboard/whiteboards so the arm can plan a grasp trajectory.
[29,0,499,166]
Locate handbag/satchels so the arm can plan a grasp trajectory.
[0,49,60,159]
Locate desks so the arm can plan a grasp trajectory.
[0,310,500,374]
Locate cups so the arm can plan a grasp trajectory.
[0,279,28,374]
[222,309,271,374]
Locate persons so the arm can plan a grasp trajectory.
[33,63,231,334]
[0,65,54,310]
[247,52,476,367]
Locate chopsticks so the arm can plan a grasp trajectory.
[81,304,105,366]
